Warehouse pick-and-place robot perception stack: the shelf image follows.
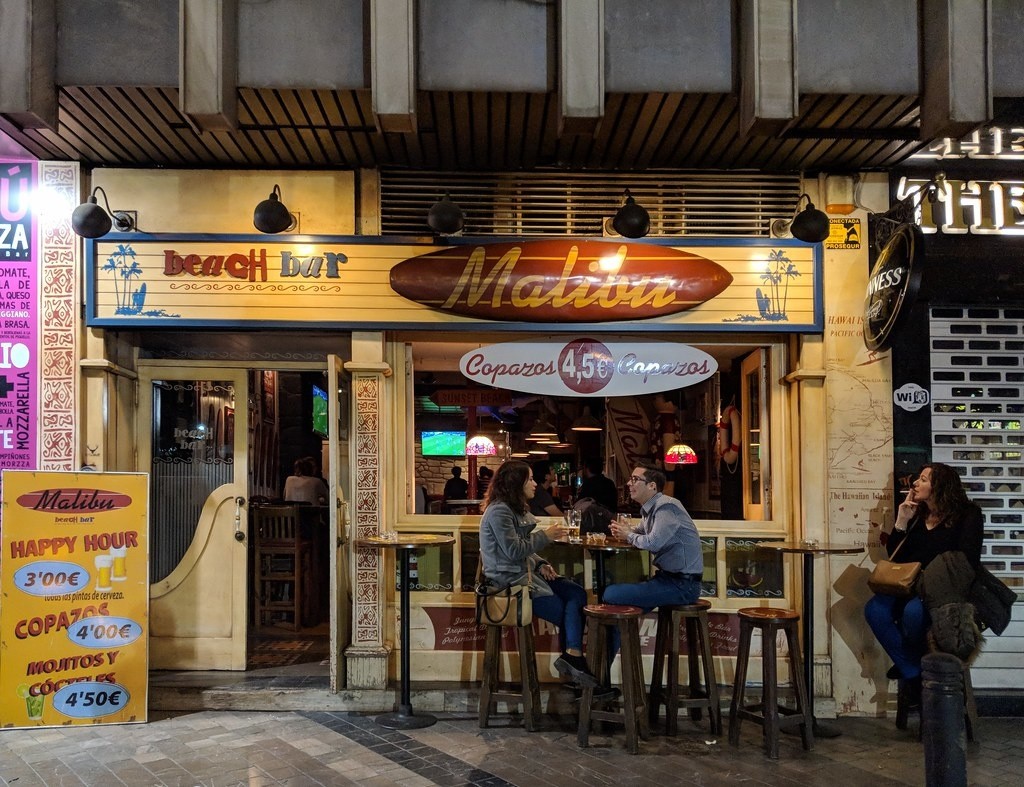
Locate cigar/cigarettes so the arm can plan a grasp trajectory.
[900,491,909,493]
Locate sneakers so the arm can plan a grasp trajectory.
[573,687,621,710]
[554,652,599,689]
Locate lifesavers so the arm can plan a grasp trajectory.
[719,405,742,465]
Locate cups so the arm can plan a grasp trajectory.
[587,532,606,543]
[25,689,44,721]
[95,545,126,592]
[568,509,582,542]
[617,513,633,542]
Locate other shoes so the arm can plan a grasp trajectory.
[906,678,921,708]
[886,664,901,680]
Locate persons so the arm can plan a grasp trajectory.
[478,466,494,499]
[526,460,563,516]
[479,460,621,700]
[579,457,618,512]
[863,462,983,712]
[603,464,703,664]
[444,466,468,515]
[283,457,329,506]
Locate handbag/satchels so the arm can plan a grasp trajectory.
[475,581,533,627]
[570,497,615,536]
[867,560,922,595]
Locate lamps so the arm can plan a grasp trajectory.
[71,187,137,239]
[466,407,498,456]
[664,390,698,464]
[511,395,603,457]
[253,185,300,235]
[770,194,830,244]
[428,192,465,236]
[604,189,650,239]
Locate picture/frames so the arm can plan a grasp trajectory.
[224,406,235,445]
[262,370,276,425]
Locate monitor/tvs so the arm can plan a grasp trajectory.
[422,429,467,457]
[307,382,330,441]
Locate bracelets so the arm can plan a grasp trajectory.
[896,525,902,530]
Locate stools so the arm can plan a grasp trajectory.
[480,624,542,733]
[894,663,980,740]
[577,605,650,755]
[729,607,814,757]
[648,598,723,736]
[254,501,314,632]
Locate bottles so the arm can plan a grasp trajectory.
[557,462,569,486]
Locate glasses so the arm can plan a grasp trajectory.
[629,476,656,490]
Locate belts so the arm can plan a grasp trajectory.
[671,574,699,581]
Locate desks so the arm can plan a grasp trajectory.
[447,499,484,504]
[266,505,329,611]
[360,534,456,730]
[553,536,646,734]
[756,543,866,738]
